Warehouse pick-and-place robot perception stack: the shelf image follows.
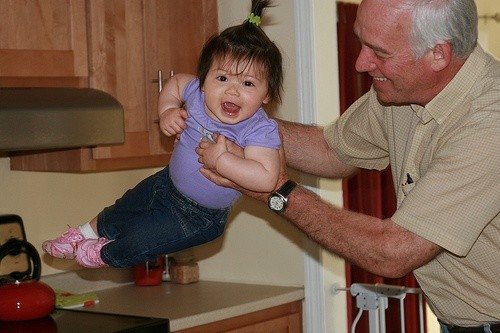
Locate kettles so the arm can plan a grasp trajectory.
[0,238,57,322]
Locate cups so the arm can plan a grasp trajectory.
[133,255,164,286]
[162,252,177,282]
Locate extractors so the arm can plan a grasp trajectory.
[1,86,126,158]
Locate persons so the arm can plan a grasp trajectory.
[43,0,282,266]
[200,0,500,333]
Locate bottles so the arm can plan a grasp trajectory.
[171,254,200,285]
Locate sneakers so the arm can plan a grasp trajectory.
[42,224,86,259]
[74,237,110,268]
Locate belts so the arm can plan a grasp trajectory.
[437,318,500,333]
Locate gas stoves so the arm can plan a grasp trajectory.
[0,214,171,333]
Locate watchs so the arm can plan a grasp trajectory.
[267,179,297,215]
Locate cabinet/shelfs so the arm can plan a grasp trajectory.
[1,0,220,174]
[173,300,303,333]
